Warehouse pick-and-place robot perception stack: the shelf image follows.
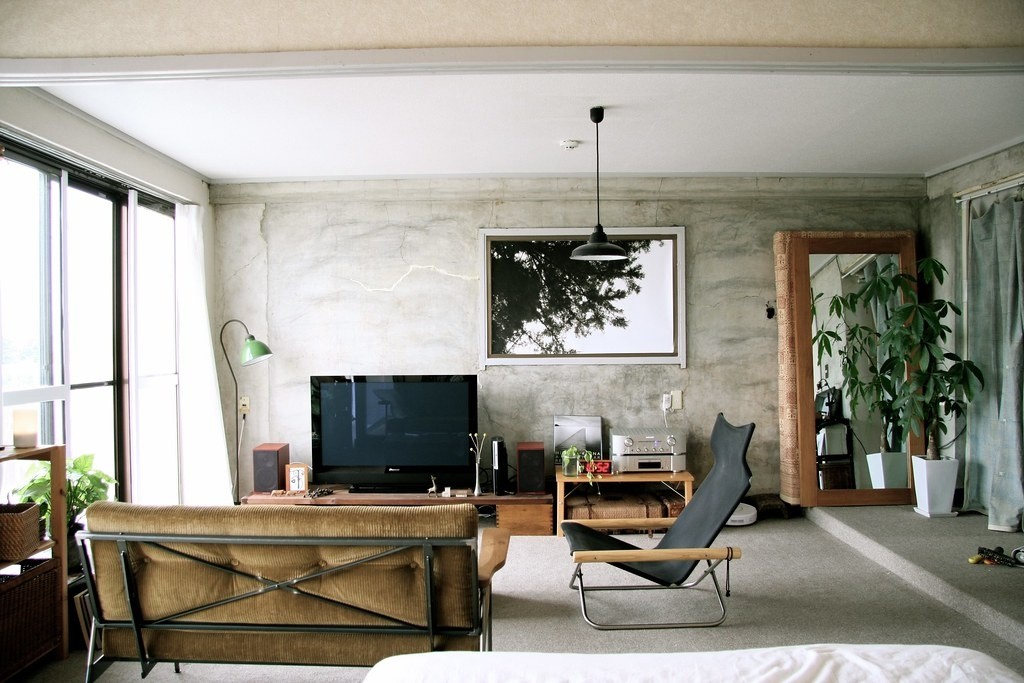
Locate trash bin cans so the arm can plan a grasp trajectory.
[817,462,853,490]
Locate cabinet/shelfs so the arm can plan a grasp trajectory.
[0,443,68,683]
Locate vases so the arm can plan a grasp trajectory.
[474,464,482,497]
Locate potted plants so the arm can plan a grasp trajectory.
[828,263,909,489]
[26,497,48,543]
[560,445,603,495]
[856,258,984,519]
[17,454,119,575]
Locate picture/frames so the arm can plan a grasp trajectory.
[284,464,308,494]
[477,227,688,371]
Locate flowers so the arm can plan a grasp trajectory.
[469,433,487,463]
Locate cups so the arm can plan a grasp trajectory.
[12,408,40,450]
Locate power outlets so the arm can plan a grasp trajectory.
[240,396,249,409]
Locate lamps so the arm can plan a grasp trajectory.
[569,106,628,261]
[220,319,272,506]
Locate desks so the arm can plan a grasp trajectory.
[555,465,694,536]
[241,490,555,536]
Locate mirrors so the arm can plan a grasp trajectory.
[789,234,927,508]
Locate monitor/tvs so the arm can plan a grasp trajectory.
[309,375,476,494]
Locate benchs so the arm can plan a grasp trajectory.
[76,500,509,683]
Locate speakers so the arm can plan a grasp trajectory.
[516,441,546,495]
[252,443,289,494]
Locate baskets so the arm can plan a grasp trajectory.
[0,558,63,682]
[0,502,39,562]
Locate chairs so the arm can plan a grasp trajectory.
[561,413,756,631]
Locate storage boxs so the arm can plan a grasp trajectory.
[663,497,685,532]
[0,503,40,562]
